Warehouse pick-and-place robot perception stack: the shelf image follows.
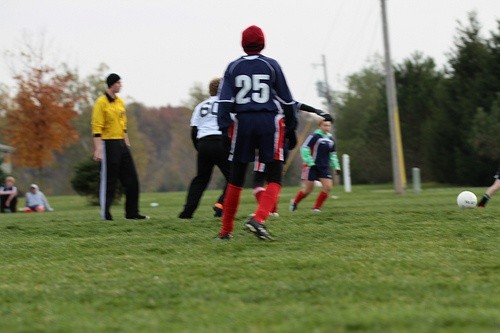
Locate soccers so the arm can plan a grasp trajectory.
[456,190,478,208]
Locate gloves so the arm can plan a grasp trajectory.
[284,130,298,151]
[221,128,232,144]
[323,114,334,124]
[335,170,340,175]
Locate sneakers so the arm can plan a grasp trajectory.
[219,233,232,239]
[244,220,275,241]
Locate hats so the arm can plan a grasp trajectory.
[106,73,120,88]
[241,25,265,55]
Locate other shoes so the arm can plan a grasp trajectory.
[178,213,193,219]
[248,212,278,216]
[125,214,146,220]
[312,208,320,212]
[290,198,297,211]
[213,205,223,217]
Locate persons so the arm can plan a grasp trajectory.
[91,73,151,221]
[288,113,344,213]
[178,78,229,220]
[17,183,55,214]
[0,176,20,214]
[247,101,335,219]
[211,25,299,242]
[476,171,500,208]
